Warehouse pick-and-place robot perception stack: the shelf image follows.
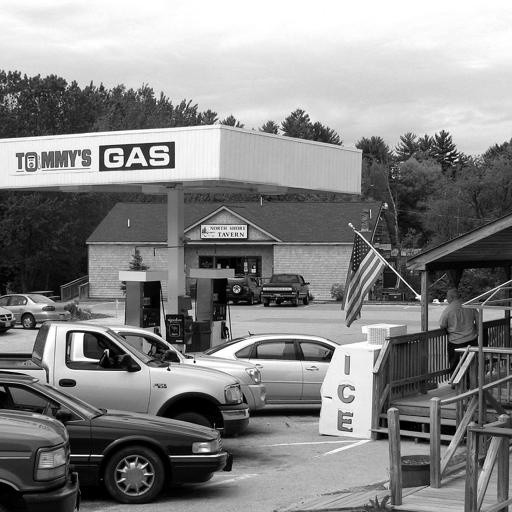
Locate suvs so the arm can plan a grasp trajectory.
[228,276,262,305]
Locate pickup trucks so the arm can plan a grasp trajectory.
[260,273,311,307]
[0,321,250,436]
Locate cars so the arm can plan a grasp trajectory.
[0,293,72,330]
[184,331,343,413]
[0,372,233,512]
[108,324,266,411]
[0,306,17,334]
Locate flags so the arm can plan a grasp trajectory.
[340,234,386,328]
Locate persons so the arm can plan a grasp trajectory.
[439,288,487,391]
